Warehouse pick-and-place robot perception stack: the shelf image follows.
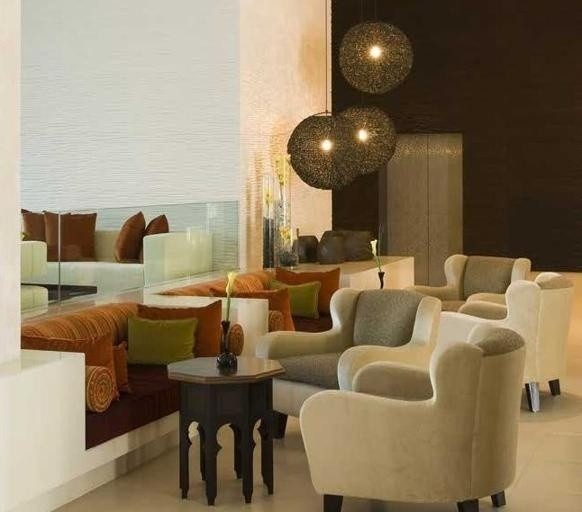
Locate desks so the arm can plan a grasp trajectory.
[22,239,48,281]
[21,285,49,318]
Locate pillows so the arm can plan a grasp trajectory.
[41,210,100,263]
[137,213,170,264]
[267,277,322,321]
[21,206,73,241]
[135,299,225,356]
[274,264,342,316]
[113,336,136,398]
[207,283,297,332]
[124,313,201,368]
[19,328,122,403]
[114,210,146,264]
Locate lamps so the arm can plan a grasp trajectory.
[336,0,417,96]
[286,22,366,193]
[337,93,399,178]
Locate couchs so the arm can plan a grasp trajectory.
[21,228,214,293]
[19,300,244,454]
[141,269,341,354]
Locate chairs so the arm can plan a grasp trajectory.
[254,286,437,443]
[402,252,532,313]
[439,270,576,416]
[294,319,528,512]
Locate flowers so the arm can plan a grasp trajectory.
[367,239,385,272]
[260,153,296,269]
[220,270,238,321]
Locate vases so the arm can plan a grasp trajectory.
[216,321,238,369]
[379,273,385,289]
[346,228,374,261]
[318,230,346,265]
[298,234,319,263]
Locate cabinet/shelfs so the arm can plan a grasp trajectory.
[291,255,415,291]
